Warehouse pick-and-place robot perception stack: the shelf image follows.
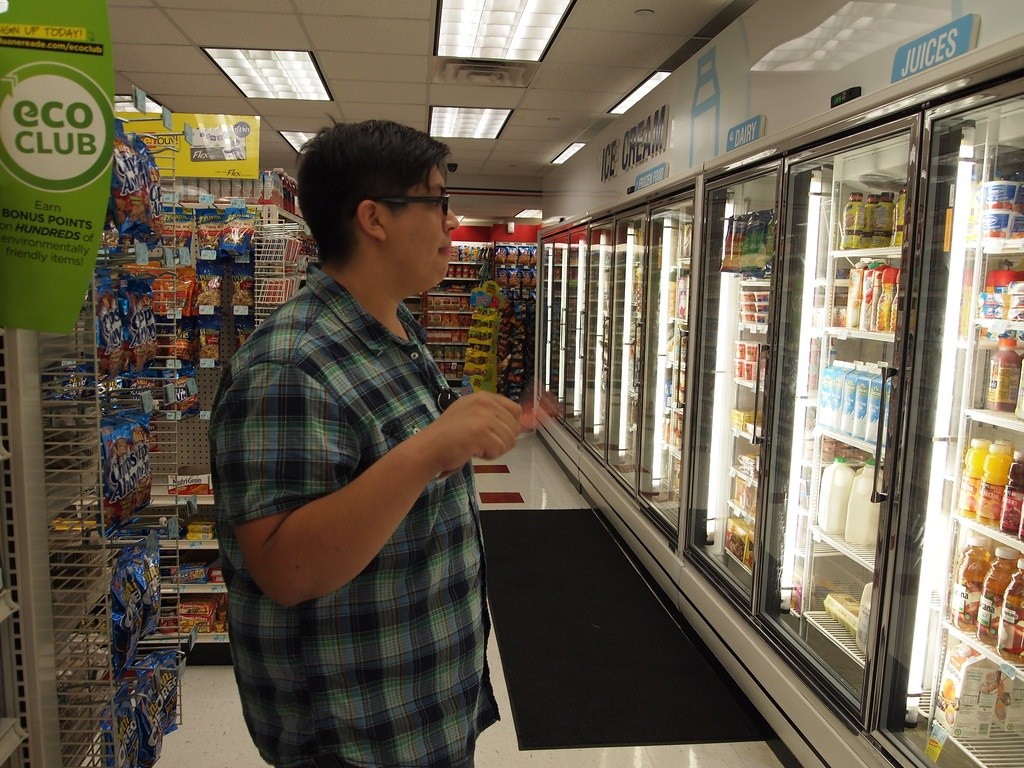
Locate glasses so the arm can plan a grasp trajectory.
[375,193,450,215]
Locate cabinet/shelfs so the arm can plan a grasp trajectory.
[0,133,198,767]
[72,204,275,667]
[402,261,486,387]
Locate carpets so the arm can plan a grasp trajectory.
[479,508,771,752]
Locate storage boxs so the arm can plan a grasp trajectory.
[283,188,295,215]
[187,521,216,540]
[177,593,228,633]
[260,236,301,262]
[168,473,214,495]
[171,561,225,584]
[175,171,283,208]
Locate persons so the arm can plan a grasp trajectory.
[208,118,522,768]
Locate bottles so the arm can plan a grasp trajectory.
[983,338,1022,414]
[972,546,1017,656]
[839,192,865,250]
[818,454,853,535]
[845,458,884,545]
[866,183,908,248]
[948,531,993,642]
[995,558,1024,666]
[958,434,1024,542]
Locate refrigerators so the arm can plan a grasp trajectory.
[532,0,1024,768]
